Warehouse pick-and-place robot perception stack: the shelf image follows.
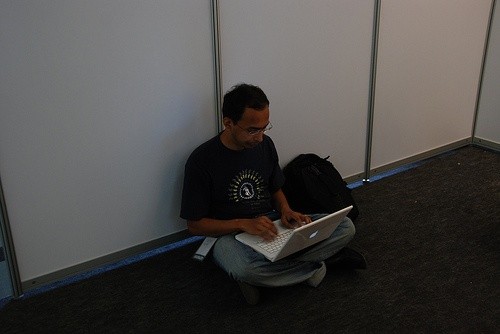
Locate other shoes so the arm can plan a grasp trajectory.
[239,282,260,304]
[324,246,354,274]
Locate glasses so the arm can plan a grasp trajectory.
[232,122,273,135]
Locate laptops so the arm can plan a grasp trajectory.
[234,205,353,262]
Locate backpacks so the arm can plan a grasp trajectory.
[282,153,360,219]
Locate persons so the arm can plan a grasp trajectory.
[181,83,368,289]
